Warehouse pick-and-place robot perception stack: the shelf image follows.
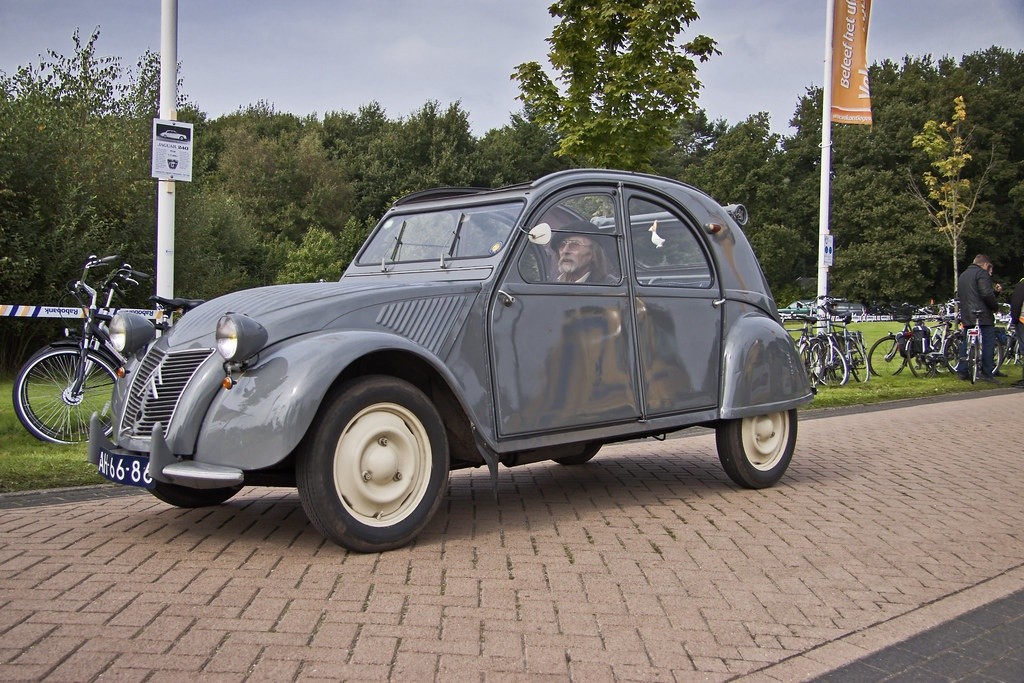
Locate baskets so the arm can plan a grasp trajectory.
[891,300,911,322]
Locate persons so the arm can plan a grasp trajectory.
[550,222,618,285]
[958,253,1002,382]
[1011,278,1024,386]
[988,265,1008,377]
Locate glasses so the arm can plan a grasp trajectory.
[559,239,590,250]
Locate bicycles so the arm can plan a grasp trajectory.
[12,255,207,444]
[790,295,870,388]
[867,302,1024,381]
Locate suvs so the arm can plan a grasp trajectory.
[777,300,817,317]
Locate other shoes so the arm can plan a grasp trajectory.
[981,375,1004,385]
[1010,380,1024,389]
[994,371,1008,377]
[958,372,969,380]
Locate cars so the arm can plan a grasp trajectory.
[90,167,817,555]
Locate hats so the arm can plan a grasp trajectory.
[549,221,607,251]
